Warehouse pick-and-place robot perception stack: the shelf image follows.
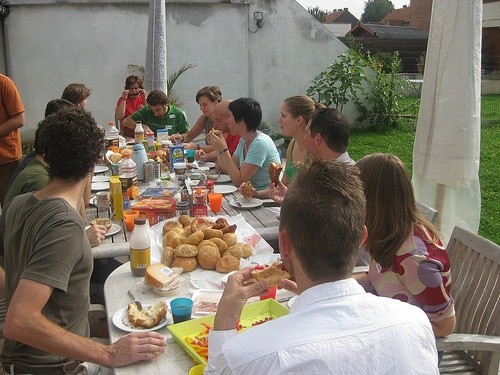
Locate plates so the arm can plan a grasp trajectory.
[91,176,109,183]
[230,198,263,208]
[91,182,110,190]
[93,166,108,172]
[213,185,237,194]
[288,295,298,308]
[199,162,215,168]
[190,289,224,318]
[112,304,169,332]
[214,174,231,182]
[85,223,121,236]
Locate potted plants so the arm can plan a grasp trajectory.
[415,55,425,73]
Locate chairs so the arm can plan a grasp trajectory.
[416,195,500,375]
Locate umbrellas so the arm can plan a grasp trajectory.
[411,0,483,249]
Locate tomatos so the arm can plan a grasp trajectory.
[185,331,209,363]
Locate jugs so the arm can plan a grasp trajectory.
[132,144,148,180]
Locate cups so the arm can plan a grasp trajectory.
[174,163,186,177]
[123,210,139,232]
[260,287,276,302]
[170,298,193,324]
[161,142,171,148]
[208,193,221,212]
[187,149,194,163]
[93,192,110,211]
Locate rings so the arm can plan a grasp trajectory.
[209,138,212,142]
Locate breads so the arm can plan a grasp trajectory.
[251,258,292,287]
[268,162,283,187]
[161,215,253,273]
[240,181,256,199]
[109,153,123,164]
[127,302,167,328]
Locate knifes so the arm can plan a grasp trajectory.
[128,290,142,309]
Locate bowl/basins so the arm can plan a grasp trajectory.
[221,271,238,285]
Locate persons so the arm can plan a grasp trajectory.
[353,152,456,367]
[207,98,282,192]
[115,75,148,135]
[238,96,326,198]
[269,107,356,204]
[0,74,25,209]
[170,85,241,174]
[122,90,190,140]
[204,156,440,375]
[0,106,168,375]
[0,84,123,338]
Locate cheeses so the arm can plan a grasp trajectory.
[145,263,177,289]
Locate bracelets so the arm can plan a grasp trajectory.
[122,98,127,100]
[181,135,185,143]
[196,144,200,150]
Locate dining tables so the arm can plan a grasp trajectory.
[84,134,282,261]
[103,261,293,375]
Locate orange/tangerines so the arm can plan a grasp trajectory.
[126,186,138,199]
[108,145,119,153]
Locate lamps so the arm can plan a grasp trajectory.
[248,12,265,33]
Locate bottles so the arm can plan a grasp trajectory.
[146,133,154,147]
[118,153,138,206]
[104,122,120,153]
[134,121,144,144]
[129,218,150,277]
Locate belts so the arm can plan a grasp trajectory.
[3,358,84,375]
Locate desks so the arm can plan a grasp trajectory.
[406,79,423,100]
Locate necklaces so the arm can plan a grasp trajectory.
[244,130,258,144]
[295,136,311,162]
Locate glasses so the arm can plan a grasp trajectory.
[128,91,141,96]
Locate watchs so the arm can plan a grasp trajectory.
[219,148,229,155]
